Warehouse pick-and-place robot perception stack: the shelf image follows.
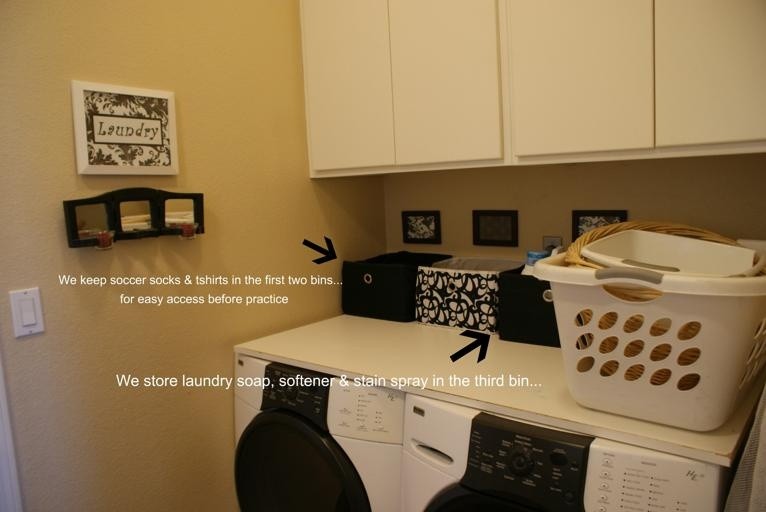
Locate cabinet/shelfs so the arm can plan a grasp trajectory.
[299,0,506,179]
[496,1,766,166]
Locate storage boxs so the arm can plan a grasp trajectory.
[498,264,561,346]
[414,256,523,333]
[341,250,452,322]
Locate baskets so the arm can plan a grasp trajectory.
[533,222,760,431]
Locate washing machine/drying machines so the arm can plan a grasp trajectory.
[402,391,722,512]
[234,353,406,512]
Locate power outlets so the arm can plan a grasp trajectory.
[543,236,562,253]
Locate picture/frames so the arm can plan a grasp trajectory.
[402,210,442,245]
[71,80,179,176]
[472,210,518,247]
[572,209,627,243]
